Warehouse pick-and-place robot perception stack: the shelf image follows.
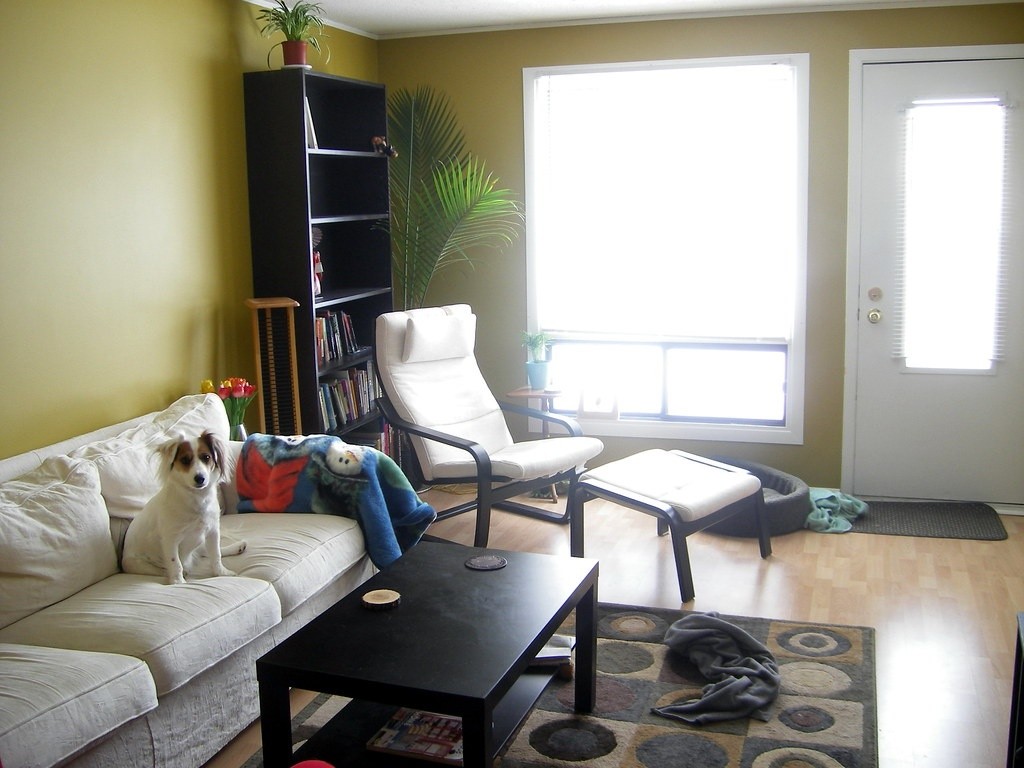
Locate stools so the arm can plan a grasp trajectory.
[570,450,773,602]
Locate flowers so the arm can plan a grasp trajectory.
[202,377,257,426]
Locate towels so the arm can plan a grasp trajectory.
[649,611,780,726]
[803,487,870,534]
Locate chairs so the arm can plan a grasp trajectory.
[373,304,602,561]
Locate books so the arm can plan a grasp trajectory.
[367,706,463,766]
[305,96,318,149]
[314,309,401,470]
[529,634,572,666]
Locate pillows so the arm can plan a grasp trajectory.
[0,454,118,629]
[72,391,231,567]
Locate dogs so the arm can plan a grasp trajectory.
[118,428,249,585]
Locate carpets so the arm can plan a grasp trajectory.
[234,605,879,768]
[851,498,1006,543]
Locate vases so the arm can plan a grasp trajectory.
[230,424,247,440]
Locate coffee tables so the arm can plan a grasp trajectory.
[255,537,598,768]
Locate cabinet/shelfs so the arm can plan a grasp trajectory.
[243,71,423,495]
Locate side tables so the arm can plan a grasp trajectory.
[505,389,562,504]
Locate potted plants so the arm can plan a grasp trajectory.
[256,0,330,68]
[519,328,557,391]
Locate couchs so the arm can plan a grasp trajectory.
[0,405,435,768]
